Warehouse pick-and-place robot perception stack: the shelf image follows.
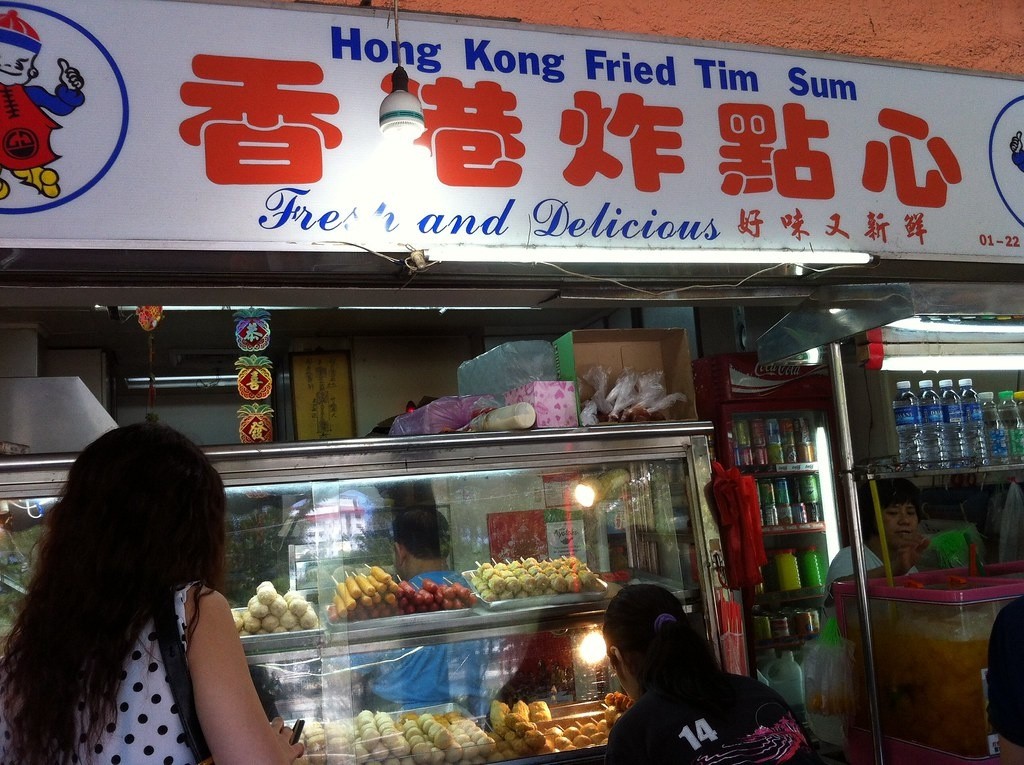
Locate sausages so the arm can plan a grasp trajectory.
[327,566,476,624]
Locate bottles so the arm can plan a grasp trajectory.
[893,381,929,471]
[917,379,943,469]
[998,390,1024,464]
[766,648,777,659]
[978,392,1012,465]
[939,379,970,468]
[1014,391,1024,423]
[762,650,809,729]
[755,545,824,593]
[957,379,989,466]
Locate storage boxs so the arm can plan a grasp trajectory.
[456,341,555,404]
[505,382,579,428]
[833,562,1024,761]
[553,328,698,426]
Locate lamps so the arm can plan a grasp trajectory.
[855,340,1024,371]
[855,318,1024,344]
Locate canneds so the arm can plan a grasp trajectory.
[729,415,823,640]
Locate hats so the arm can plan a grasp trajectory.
[984,594,1024,748]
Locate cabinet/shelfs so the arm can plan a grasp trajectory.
[0,425,715,765]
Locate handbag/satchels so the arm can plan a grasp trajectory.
[148,587,305,765]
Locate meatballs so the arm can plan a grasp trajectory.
[286,706,626,765]
[470,556,605,602]
[230,581,317,636]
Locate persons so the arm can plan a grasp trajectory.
[603,584,823,765]
[485,591,612,733]
[325,505,494,718]
[988,594,1024,765]
[822,478,930,629]
[0,422,304,765]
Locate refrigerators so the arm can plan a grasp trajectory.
[693,352,852,738]
